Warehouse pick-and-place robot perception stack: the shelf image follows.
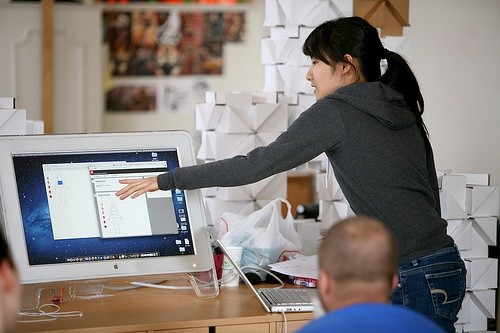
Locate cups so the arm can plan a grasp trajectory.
[221,247,245,287]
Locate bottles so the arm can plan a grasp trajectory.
[202,224,224,284]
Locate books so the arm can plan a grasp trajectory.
[287,275,318,288]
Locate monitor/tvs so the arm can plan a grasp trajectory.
[0,129,219,316]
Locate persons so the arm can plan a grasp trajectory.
[115,17,467,333]
[294,216,446,333]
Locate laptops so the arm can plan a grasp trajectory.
[216,239,319,312]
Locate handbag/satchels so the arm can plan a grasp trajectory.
[219,197,303,281]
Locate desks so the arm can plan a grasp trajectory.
[1,273,317,333]
